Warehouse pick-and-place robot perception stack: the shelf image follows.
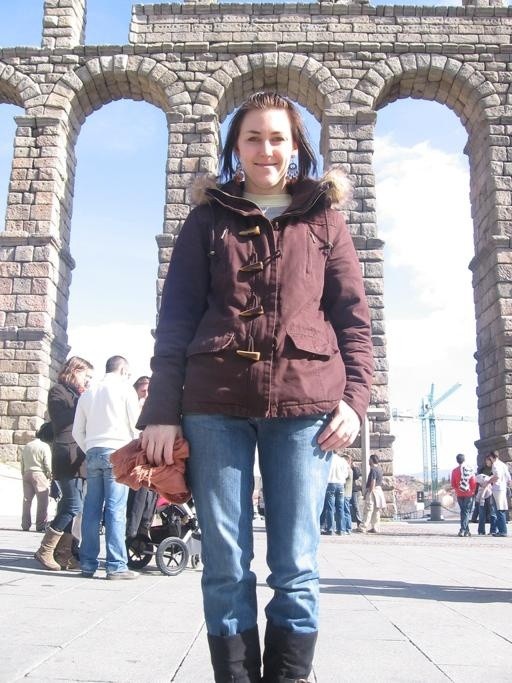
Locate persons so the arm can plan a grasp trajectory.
[136,92,374,683]
[318,450,386,536]
[452,450,512,536]
[21,356,158,580]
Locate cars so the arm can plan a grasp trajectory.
[257,486,266,520]
[100,506,105,535]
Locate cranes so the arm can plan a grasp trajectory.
[420,380,466,503]
[391,398,464,502]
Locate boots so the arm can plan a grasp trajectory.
[261,627,318,683]
[53,532,83,569]
[34,525,65,571]
[207,625,261,683]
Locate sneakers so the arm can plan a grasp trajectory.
[22,524,47,533]
[320,525,379,535]
[81,569,95,578]
[458,529,508,538]
[106,568,141,581]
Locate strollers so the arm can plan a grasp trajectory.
[127,489,202,577]
